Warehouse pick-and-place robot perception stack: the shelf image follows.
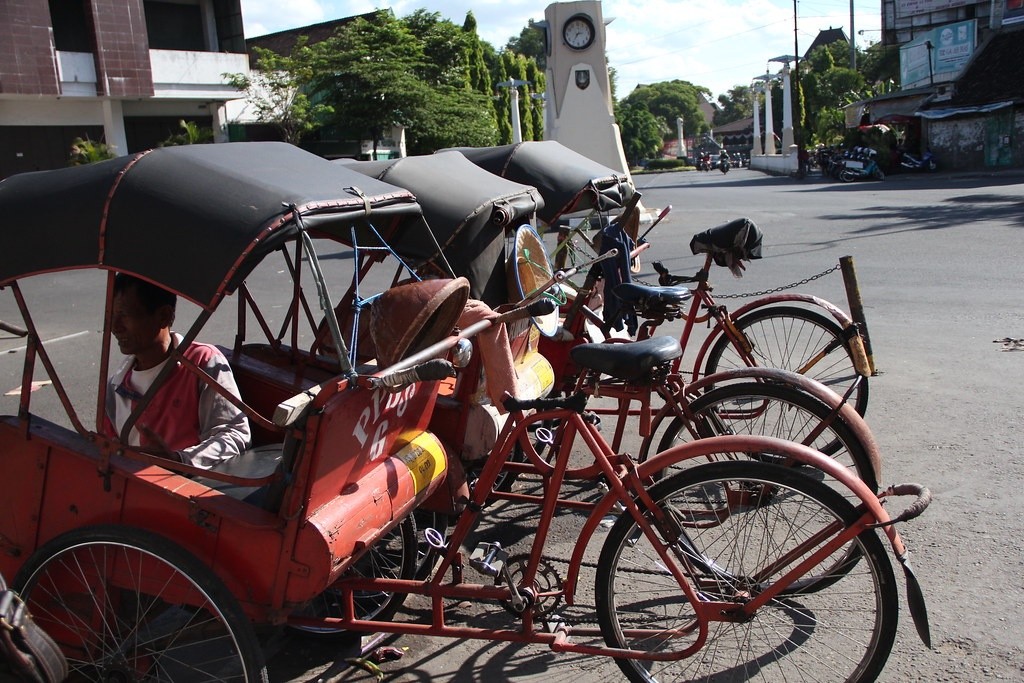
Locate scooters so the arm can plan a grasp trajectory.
[817,143,885,183]
[695,159,712,172]
[719,157,730,175]
[731,157,746,168]
[900,146,938,175]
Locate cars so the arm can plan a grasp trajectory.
[710,155,721,169]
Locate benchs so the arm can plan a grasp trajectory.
[189,349,449,611]
[538,242,651,391]
[428,304,557,462]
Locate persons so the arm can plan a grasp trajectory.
[718,150,729,164]
[804,146,817,172]
[700,151,712,170]
[818,144,825,159]
[105,272,251,471]
[731,153,747,167]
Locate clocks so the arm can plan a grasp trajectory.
[562,15,596,50]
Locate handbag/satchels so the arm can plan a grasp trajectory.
[0,589,67,683]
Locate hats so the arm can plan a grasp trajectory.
[503,222,560,337]
[590,207,641,273]
[370,277,470,365]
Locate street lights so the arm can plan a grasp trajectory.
[496,79,531,143]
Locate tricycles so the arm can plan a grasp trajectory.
[0,140,932,683]
